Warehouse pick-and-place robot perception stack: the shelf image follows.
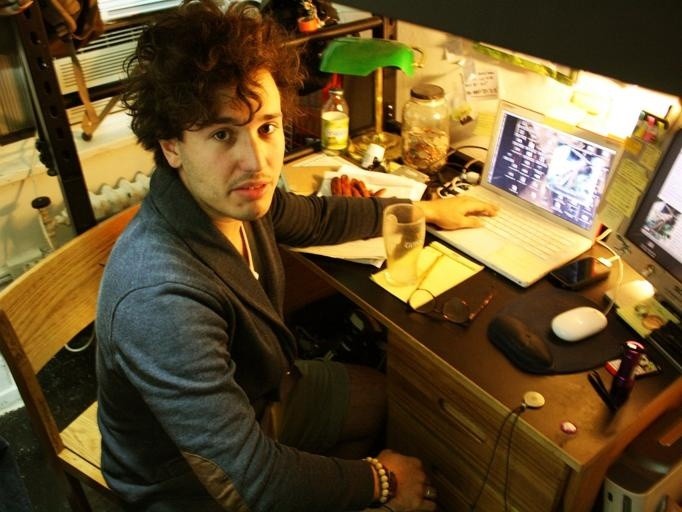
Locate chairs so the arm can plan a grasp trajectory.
[0,200,328,511]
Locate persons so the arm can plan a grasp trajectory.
[92,0,502,510]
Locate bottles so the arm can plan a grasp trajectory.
[321,88,350,152]
[400,83,451,174]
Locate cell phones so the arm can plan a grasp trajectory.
[549,257,610,291]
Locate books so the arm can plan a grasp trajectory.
[369,240,486,311]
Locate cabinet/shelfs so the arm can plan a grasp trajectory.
[1,3,396,388]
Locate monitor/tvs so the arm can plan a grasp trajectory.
[605,126,682,312]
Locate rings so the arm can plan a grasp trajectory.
[425,485,431,497]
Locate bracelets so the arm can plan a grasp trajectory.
[370,462,398,510]
[362,455,390,504]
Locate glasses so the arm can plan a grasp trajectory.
[406,255,495,323]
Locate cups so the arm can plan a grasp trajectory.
[381,202,427,287]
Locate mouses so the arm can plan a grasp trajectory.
[551,307,608,342]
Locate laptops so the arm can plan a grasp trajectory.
[426,99,625,287]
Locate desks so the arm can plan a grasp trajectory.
[217,126,682,512]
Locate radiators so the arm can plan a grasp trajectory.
[52,1,186,95]
